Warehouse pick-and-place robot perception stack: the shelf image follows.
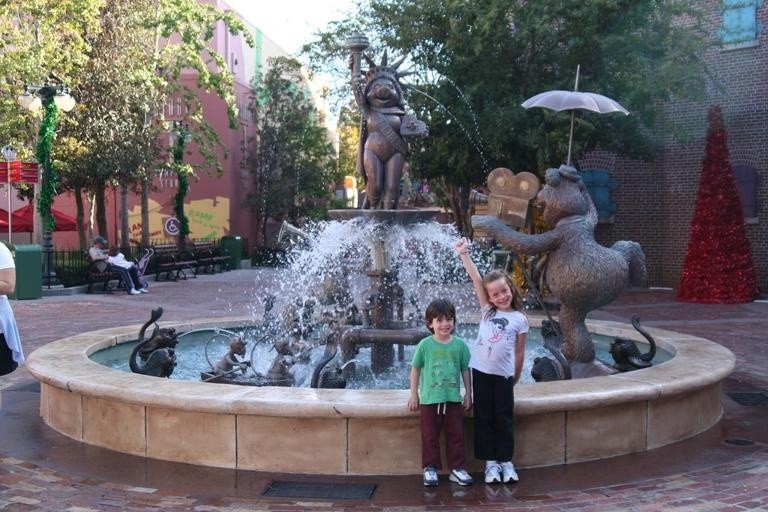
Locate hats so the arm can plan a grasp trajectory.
[94,235,108,244]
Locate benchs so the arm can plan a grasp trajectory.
[153,243,232,281]
[80,247,148,294]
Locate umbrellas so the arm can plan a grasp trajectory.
[519,87,630,164]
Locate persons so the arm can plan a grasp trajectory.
[87,236,149,295]
[106,244,149,293]
[454,233,529,487]
[0,240,25,377]
[405,297,476,490]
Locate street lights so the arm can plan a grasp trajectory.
[168,119,195,247]
[15,66,78,284]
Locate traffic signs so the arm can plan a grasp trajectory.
[0,163,40,182]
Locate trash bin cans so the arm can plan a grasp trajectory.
[221,235,242,269]
[14,244,43,299]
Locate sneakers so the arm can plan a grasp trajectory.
[500,461,520,484]
[138,288,148,294]
[484,460,502,483]
[422,464,439,486]
[448,468,473,486]
[129,288,141,295]
